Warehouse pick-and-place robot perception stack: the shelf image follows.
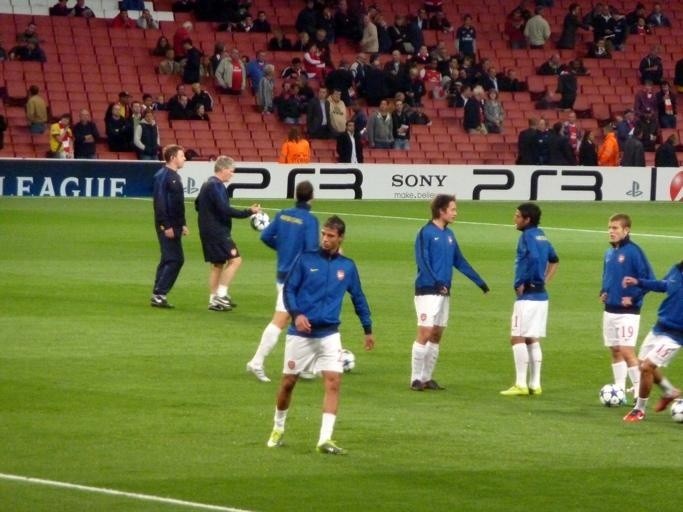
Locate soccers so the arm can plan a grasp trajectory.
[671,399,683,422]
[344,347,356,373]
[250,213,269,231]
[600,383,624,406]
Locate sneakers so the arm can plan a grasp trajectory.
[151,302,175,309]
[424,380,445,390]
[500,386,529,397]
[410,380,423,391]
[208,304,232,311]
[213,296,236,308]
[151,296,167,304]
[267,428,284,447]
[623,409,645,421]
[529,387,542,396]
[655,388,680,411]
[316,440,348,455]
[247,361,271,383]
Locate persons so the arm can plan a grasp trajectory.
[194,155,261,313]
[499,203,559,398]
[598,213,658,408]
[103,92,133,134]
[149,143,190,310]
[155,1,683,166]
[50,0,70,16]
[67,0,95,18]
[136,9,159,29]
[246,181,322,383]
[106,104,128,152]
[408,192,492,392]
[140,93,155,119]
[266,215,376,456]
[124,101,143,152]
[49,113,73,159]
[111,9,135,29]
[7,38,46,62]
[72,109,99,159]
[16,23,41,43]
[133,109,160,162]
[619,260,683,423]
[24,85,47,133]
